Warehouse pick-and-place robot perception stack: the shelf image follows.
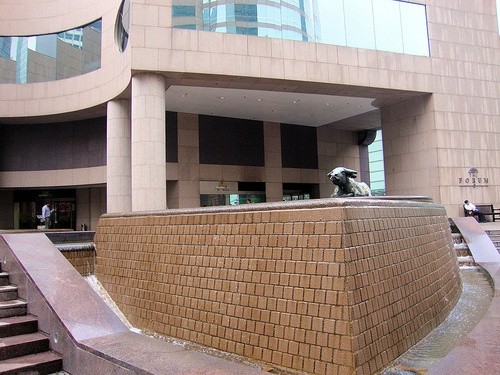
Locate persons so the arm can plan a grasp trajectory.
[41,201,54,228]
[464,199,486,222]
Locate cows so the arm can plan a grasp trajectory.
[327,167,371,197]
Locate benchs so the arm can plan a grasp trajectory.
[463,204,500,223]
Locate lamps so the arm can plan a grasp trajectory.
[216,181,230,191]
